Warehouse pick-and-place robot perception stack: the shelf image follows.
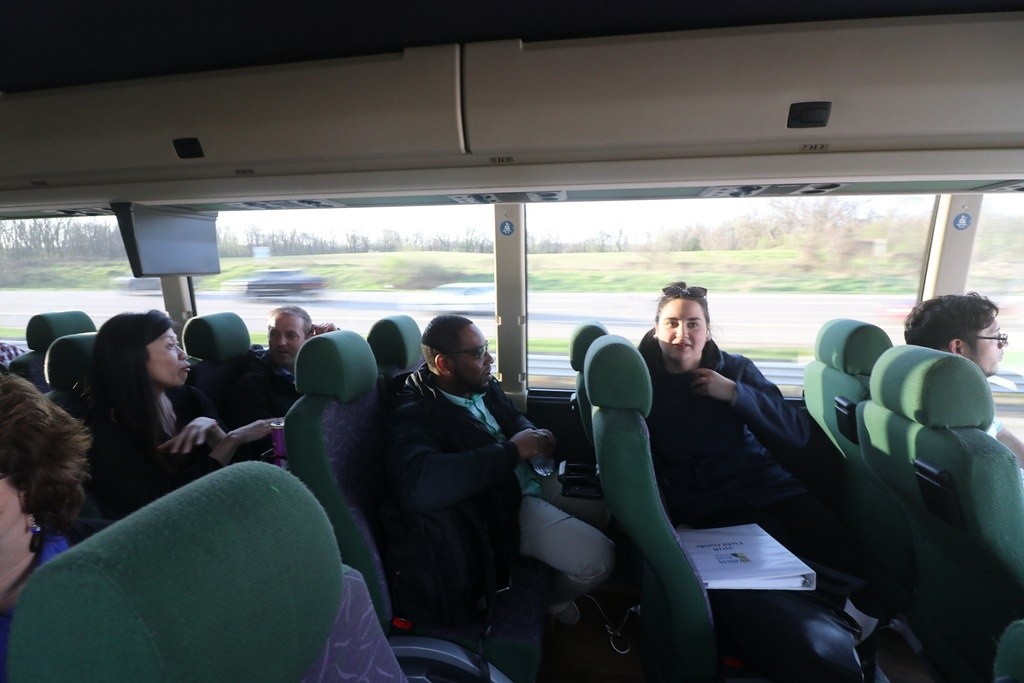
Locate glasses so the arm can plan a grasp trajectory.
[662,281,707,297]
[964,332,1008,346]
[456,340,488,360]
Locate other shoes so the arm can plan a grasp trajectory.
[559,601,579,623]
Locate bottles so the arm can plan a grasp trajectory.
[529,452,555,477]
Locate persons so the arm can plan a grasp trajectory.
[242,306,340,417]
[905,293,1024,472]
[638,281,890,683]
[0,371,92,683]
[91,310,282,521]
[362,315,616,625]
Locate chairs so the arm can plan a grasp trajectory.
[282,316,543,683]
[803,319,914,613]
[6,460,411,683]
[9,310,99,413]
[856,344,1023,683]
[570,320,892,683]
[181,314,262,398]
